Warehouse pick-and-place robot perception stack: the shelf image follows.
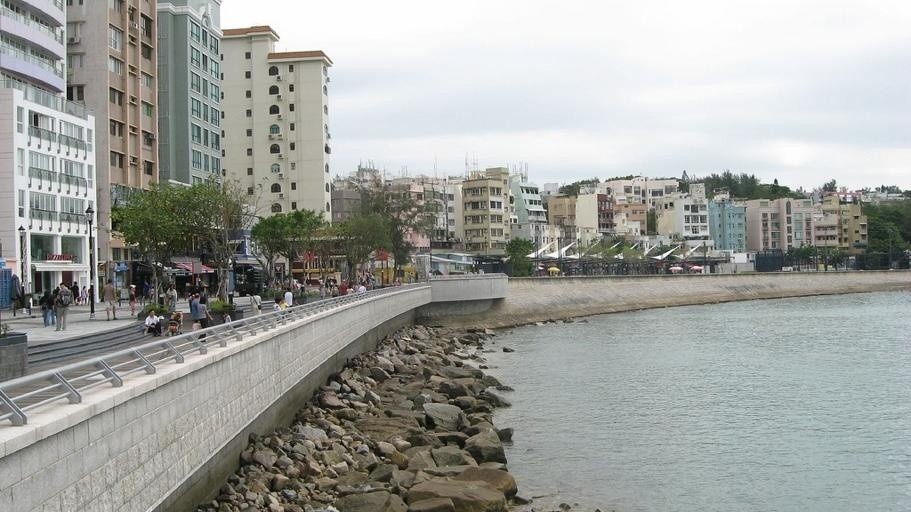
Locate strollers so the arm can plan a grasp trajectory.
[167,311,183,336]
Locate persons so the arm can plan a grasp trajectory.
[143,272,378,343]
[127,284,138,316]
[40,280,91,330]
[102,279,118,321]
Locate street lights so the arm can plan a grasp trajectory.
[86,204,96,320]
[18,226,26,314]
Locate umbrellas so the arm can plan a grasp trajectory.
[668,266,685,274]
[691,266,704,271]
[10,274,24,315]
[548,266,561,277]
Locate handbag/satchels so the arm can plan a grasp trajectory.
[258,305,262,310]
